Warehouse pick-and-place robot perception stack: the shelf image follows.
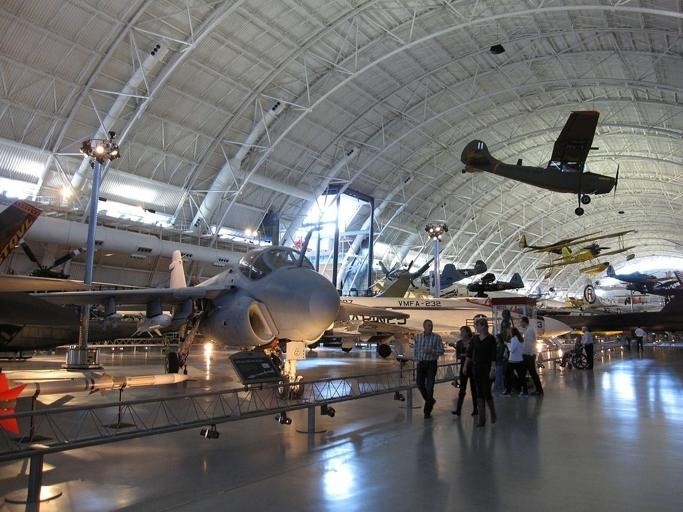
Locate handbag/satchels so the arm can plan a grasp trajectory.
[462,354,479,376]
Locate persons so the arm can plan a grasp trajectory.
[515,317,544,396]
[412,319,444,420]
[499,328,524,398]
[461,317,497,427]
[581,326,594,369]
[559,335,583,368]
[634,325,646,354]
[451,326,478,417]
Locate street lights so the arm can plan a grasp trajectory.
[421,218,448,298]
[65,129,121,370]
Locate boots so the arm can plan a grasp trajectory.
[476,397,486,428]
[486,399,497,424]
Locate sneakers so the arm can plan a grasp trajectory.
[498,388,545,398]
[451,410,461,417]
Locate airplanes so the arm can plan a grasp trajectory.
[422,260,486,291]
[604,264,682,300]
[457,110,621,215]
[366,249,445,302]
[307,291,574,348]
[516,227,637,279]
[311,316,410,358]
[465,271,523,300]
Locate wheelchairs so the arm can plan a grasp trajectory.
[566,344,588,370]
